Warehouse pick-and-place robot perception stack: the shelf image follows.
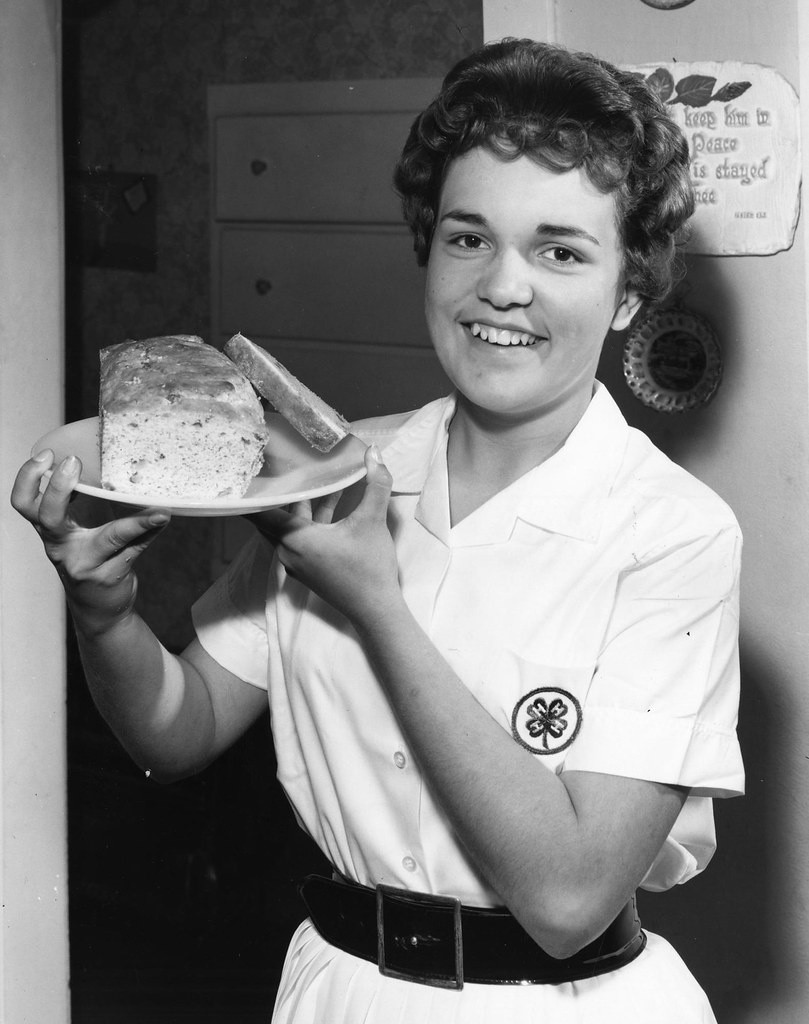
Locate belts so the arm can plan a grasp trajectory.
[295,859,649,997]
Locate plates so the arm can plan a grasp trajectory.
[31,413,371,518]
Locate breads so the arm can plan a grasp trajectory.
[223,333,353,453]
[100,334,269,502]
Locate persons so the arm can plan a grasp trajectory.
[11,38,746,1024]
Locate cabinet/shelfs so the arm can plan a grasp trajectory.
[206,77,456,422]
